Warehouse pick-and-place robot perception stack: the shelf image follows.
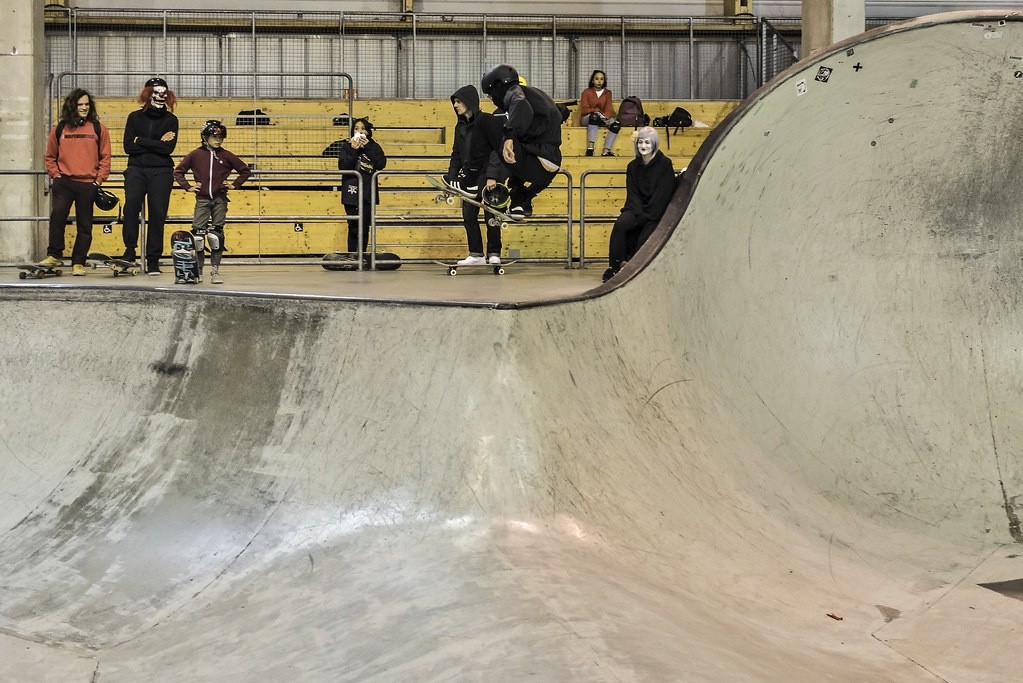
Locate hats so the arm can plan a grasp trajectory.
[145,77,167,88]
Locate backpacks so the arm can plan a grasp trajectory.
[616,96,645,127]
[666,107,692,127]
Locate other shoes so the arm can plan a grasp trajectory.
[586,147,593,156]
[601,152,614,157]
[602,259,629,283]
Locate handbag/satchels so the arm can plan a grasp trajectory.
[95,188,119,211]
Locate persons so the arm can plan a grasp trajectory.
[482,62,565,221]
[175,119,253,284]
[119,76,180,277]
[442,84,504,266]
[580,68,621,156]
[336,118,388,254]
[31,87,113,280]
[599,126,676,284]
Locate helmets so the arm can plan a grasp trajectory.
[518,75,527,86]
[481,64,519,93]
[201,119,227,144]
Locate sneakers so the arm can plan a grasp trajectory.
[210,272,224,283]
[198,273,203,281]
[442,174,478,199]
[121,248,137,267]
[37,256,64,267]
[489,256,501,264]
[503,206,526,220]
[146,256,162,275]
[457,255,488,265]
[72,264,86,276]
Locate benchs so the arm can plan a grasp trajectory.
[46,88,749,260]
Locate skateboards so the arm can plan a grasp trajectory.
[321,248,402,271]
[424,172,527,229]
[15,260,67,280]
[433,260,517,275]
[170,230,200,284]
[88,252,138,276]
